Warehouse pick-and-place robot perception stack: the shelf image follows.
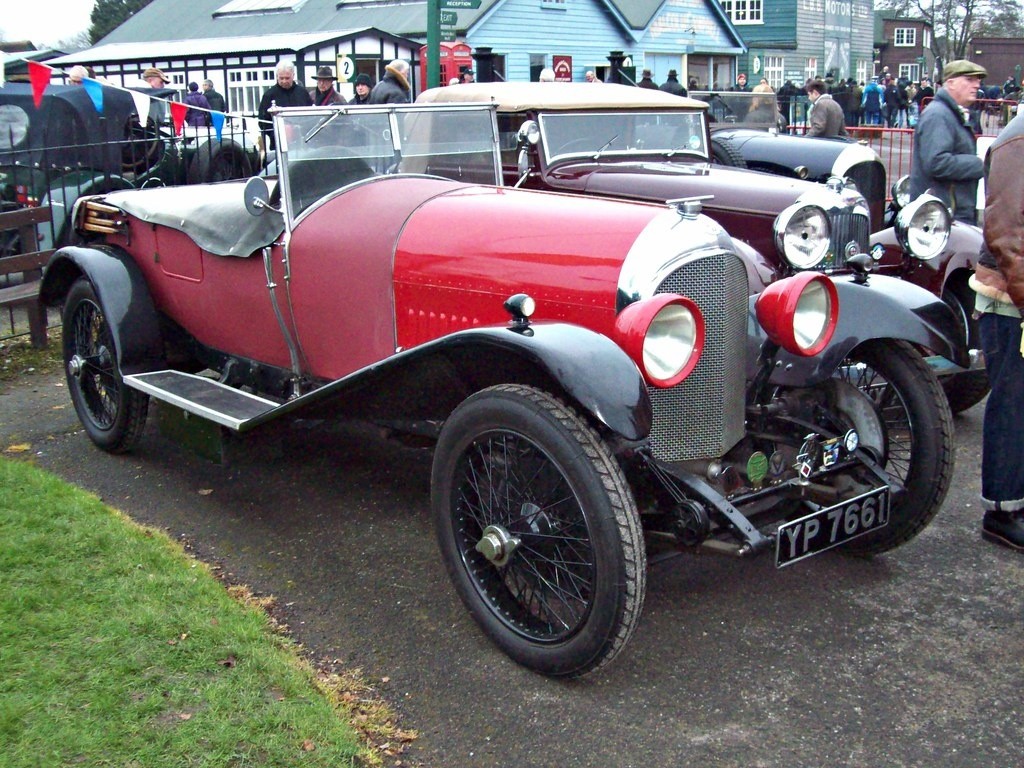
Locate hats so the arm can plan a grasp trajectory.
[311,67,338,80]
[738,74,746,79]
[668,70,678,75]
[144,68,171,84]
[355,74,373,87]
[189,82,199,92]
[826,73,833,77]
[464,69,474,75]
[943,60,987,81]
[642,69,652,78]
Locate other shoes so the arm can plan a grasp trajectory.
[981,512,1024,550]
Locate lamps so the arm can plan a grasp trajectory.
[685,28,697,36]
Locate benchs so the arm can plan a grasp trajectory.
[0,207,56,348]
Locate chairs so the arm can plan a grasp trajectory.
[268,145,378,211]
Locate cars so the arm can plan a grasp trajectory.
[39,95,957,678]
[0,84,261,273]
[385,81,983,414]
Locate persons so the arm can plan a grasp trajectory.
[658,69,687,125]
[183,80,225,126]
[68,65,96,86]
[347,74,372,146]
[970,75,1024,134]
[459,68,476,84]
[369,59,412,173]
[825,66,933,129]
[760,75,822,134]
[309,66,348,146]
[969,113,1024,550]
[257,59,313,150]
[539,69,555,82]
[744,84,786,134]
[910,60,987,225]
[636,69,659,125]
[143,68,171,122]
[726,74,752,122]
[805,80,848,137]
[586,71,602,83]
